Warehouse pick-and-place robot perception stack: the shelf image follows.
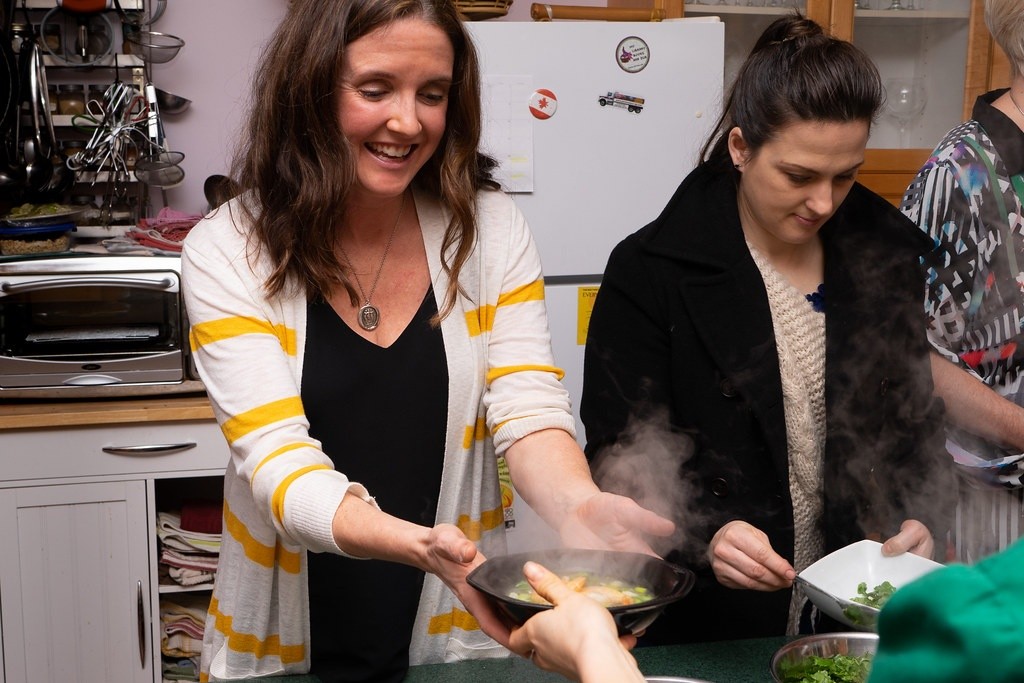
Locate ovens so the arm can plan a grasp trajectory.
[0,223,190,389]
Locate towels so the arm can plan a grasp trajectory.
[155,496,238,683]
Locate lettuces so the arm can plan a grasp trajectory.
[777,580,899,682]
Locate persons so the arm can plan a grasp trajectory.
[894,0,1024,563]
[175,0,680,683]
[505,529,1023,683]
[576,11,961,650]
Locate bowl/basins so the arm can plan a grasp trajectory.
[768,629,880,683]
[1,224,78,257]
[795,536,952,630]
[466,548,695,637]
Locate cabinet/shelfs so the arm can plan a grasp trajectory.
[606,0,1012,215]
[0,394,235,683]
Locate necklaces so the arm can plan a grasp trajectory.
[327,188,408,330]
[1009,89,1024,115]
